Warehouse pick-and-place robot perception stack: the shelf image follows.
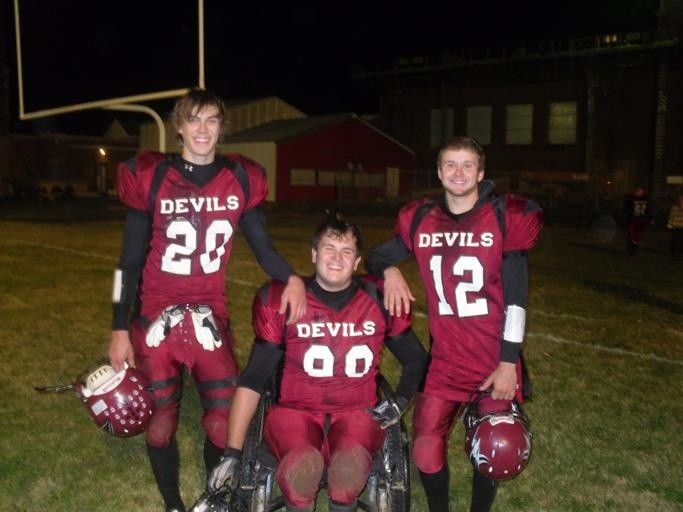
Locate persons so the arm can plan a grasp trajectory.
[361,134,543,512]
[206,217,430,511]
[107,88,309,512]
[621,185,655,265]
[666,194,682,257]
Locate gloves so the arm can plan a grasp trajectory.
[365,391,411,430]
[144,303,184,348]
[192,304,222,351]
[207,447,244,495]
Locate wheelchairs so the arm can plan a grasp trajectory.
[232,361,411,512]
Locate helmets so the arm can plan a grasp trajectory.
[463,386,533,482]
[77,358,153,436]
[169,489,251,512]
